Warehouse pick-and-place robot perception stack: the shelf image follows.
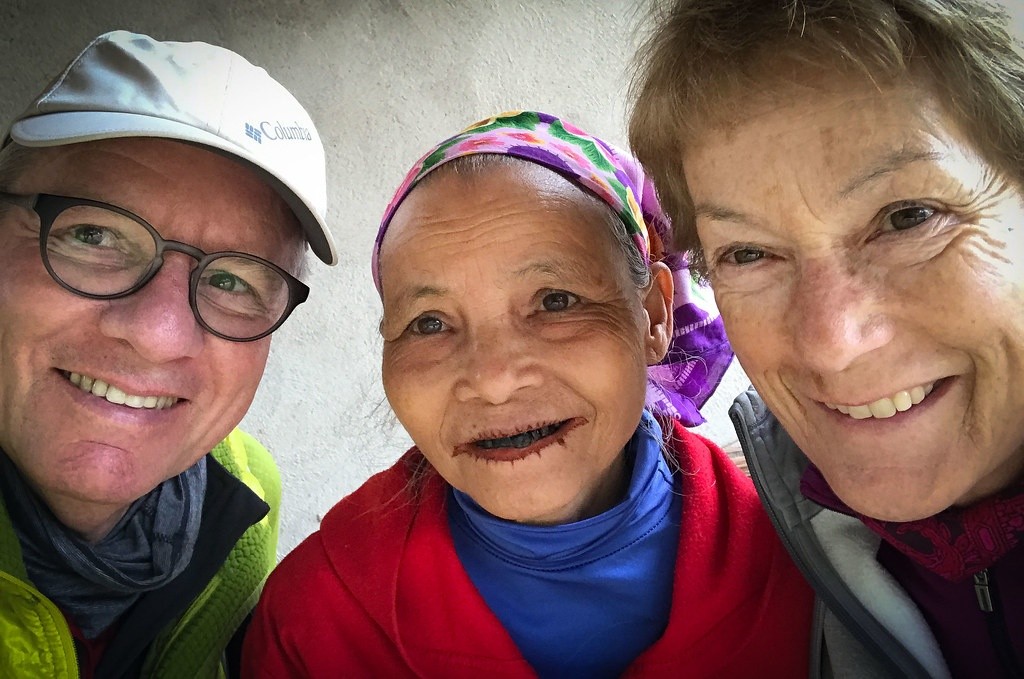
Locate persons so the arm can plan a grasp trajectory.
[1,29,336,679]
[623,1,1024,678]
[235,111,821,678]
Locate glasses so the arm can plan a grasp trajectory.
[0,192,310,342]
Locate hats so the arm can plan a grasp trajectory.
[0,30,339,267]
